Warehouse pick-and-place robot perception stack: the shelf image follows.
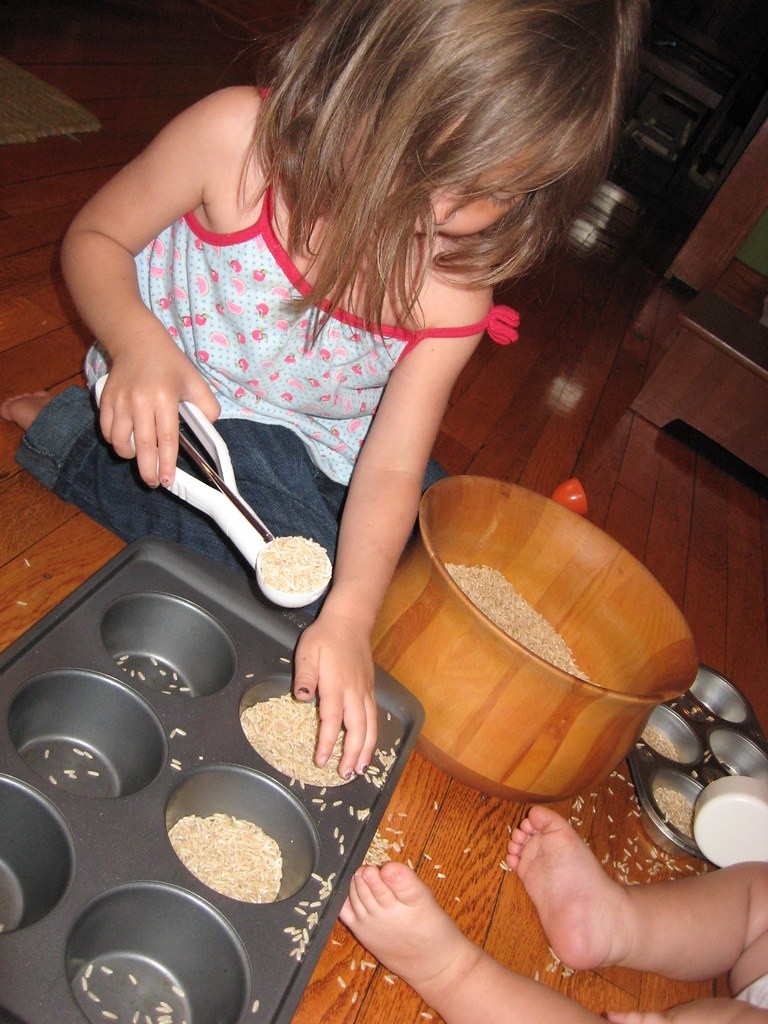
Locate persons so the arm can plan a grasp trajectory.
[0,0,650,782]
[340,806,768,1024]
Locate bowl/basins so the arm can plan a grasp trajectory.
[366,475,699,803]
